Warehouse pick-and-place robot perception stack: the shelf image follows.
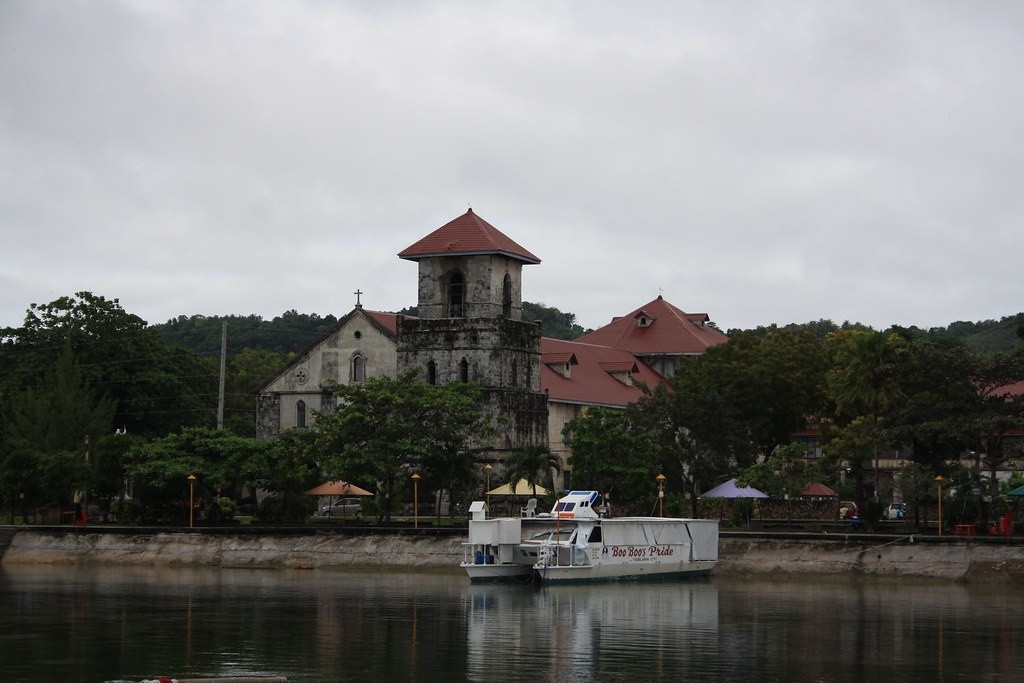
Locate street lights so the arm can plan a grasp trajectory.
[657,474,666,516]
[410,473,421,528]
[484,462,493,517]
[933,474,945,537]
[186,474,197,528]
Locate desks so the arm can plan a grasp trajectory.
[61,510,78,525]
[955,524,975,535]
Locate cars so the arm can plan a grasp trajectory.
[322,497,368,516]
[840,500,860,521]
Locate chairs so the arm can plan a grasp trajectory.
[520,498,538,517]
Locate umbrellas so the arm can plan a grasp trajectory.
[1006,484,1024,496]
[696,478,769,498]
[798,482,836,497]
[485,478,552,517]
[301,480,374,518]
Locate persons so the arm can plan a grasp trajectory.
[192,501,205,519]
[72,503,86,526]
[1007,462,1017,467]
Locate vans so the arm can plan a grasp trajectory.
[887,503,907,520]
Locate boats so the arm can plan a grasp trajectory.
[459,494,601,581]
[532,515,720,582]
